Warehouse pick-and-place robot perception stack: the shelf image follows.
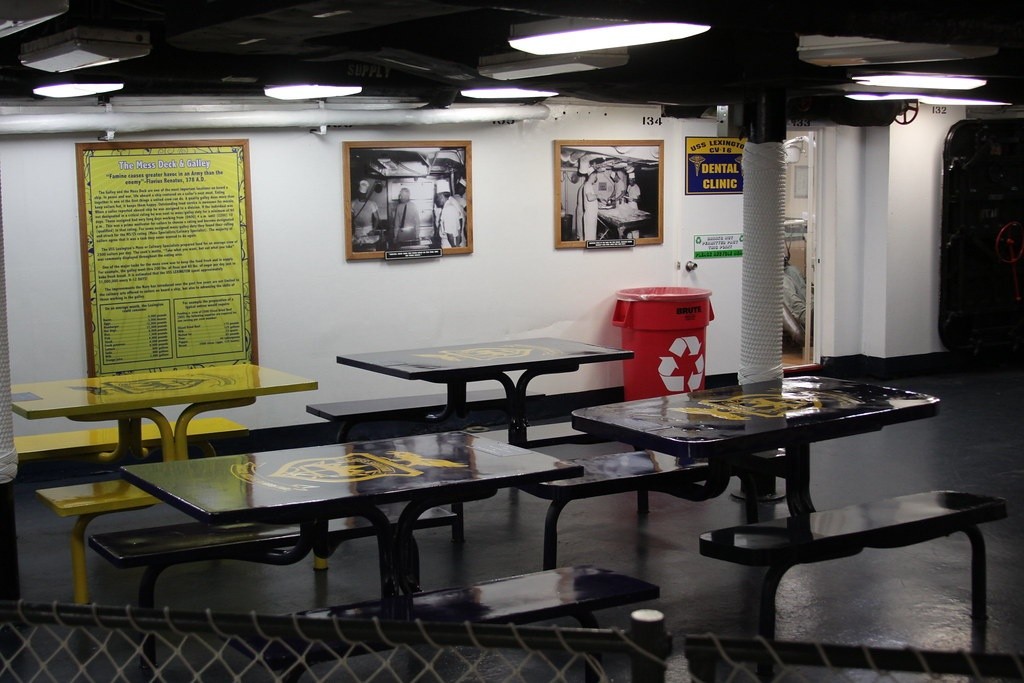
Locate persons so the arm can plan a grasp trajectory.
[393,188,420,245]
[576,172,602,241]
[608,171,626,234]
[623,173,640,238]
[351,180,379,251]
[434,179,467,248]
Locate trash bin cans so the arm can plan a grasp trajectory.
[612,286,715,400]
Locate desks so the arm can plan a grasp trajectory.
[571,374,943,508]
[335,338,640,516]
[112,429,585,597]
[10,364,320,461]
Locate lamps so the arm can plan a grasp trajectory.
[18,17,1013,106]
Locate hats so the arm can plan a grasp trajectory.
[629,173,635,179]
[459,179,467,187]
[610,171,616,179]
[587,167,595,176]
[359,180,369,194]
[435,180,451,194]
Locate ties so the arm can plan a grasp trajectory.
[400,204,407,232]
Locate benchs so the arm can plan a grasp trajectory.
[20,386,1012,651]
